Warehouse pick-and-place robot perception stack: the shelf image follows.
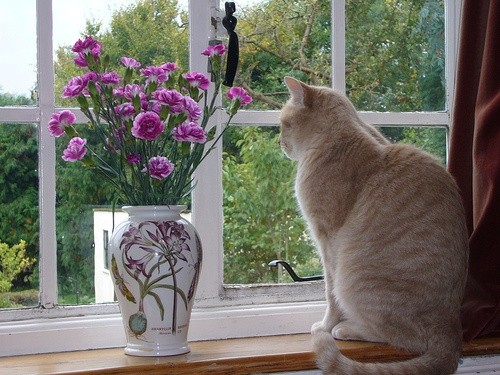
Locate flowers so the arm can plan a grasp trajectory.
[47,31,261,206]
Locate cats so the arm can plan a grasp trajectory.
[276,75,470,369]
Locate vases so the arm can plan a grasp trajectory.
[106,207,204,355]
